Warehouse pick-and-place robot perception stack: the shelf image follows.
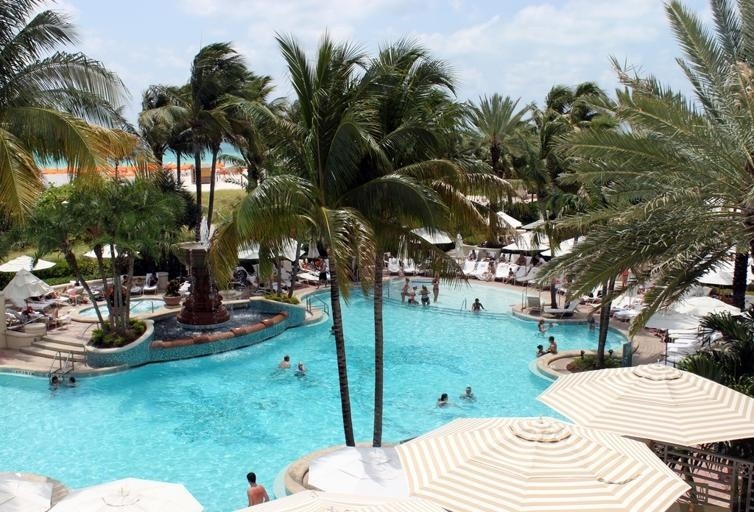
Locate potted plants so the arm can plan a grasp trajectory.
[161,278,182,305]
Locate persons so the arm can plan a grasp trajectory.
[1,274,266,391]
[276,249,601,403]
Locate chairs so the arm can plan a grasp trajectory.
[659,324,715,368]
[385,253,642,321]
[0,271,159,332]
[232,257,332,295]
